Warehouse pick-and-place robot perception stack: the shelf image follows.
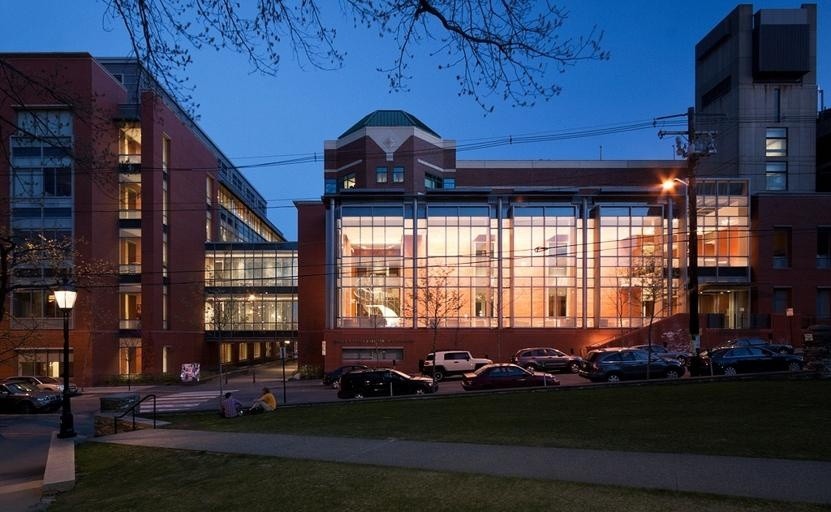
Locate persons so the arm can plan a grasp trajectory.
[248,386,277,413]
[181,363,200,383]
[219,392,244,418]
[192,371,200,385]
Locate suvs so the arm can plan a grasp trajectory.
[511,347,585,374]
[418,349,494,383]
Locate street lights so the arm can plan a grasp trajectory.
[51,275,79,440]
[661,177,701,354]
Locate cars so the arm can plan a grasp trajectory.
[6,375,81,398]
[321,364,368,390]
[336,368,439,400]
[0,378,63,414]
[631,343,690,366]
[685,344,809,376]
[708,335,796,354]
[459,362,562,392]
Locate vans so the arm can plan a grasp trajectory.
[578,347,685,383]
